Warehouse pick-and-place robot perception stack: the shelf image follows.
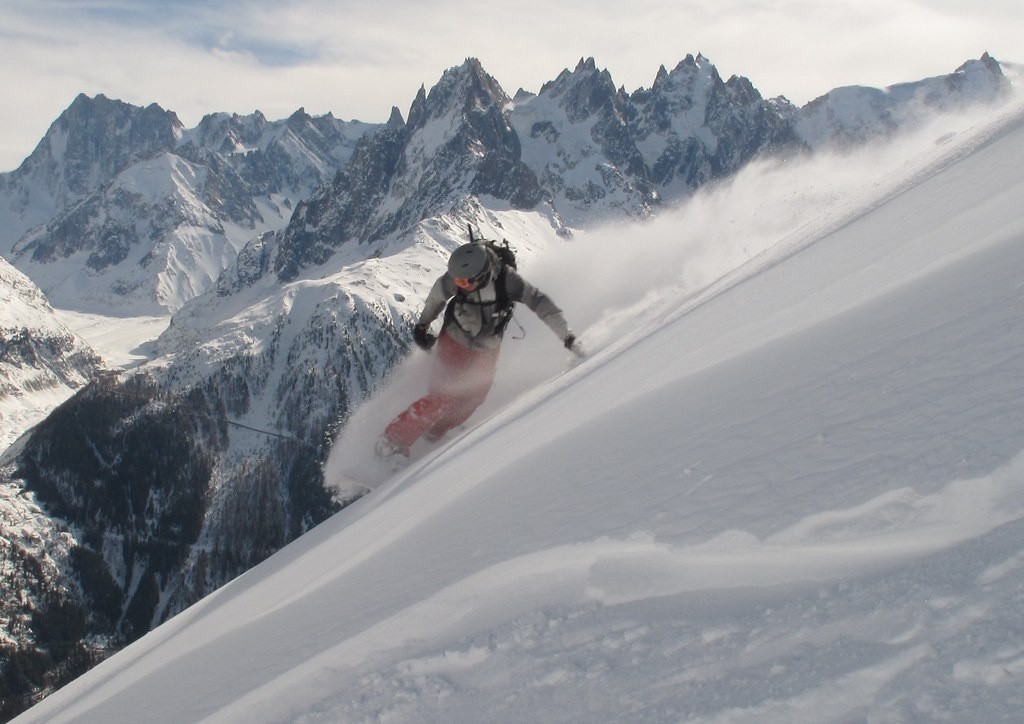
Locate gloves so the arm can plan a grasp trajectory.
[564,336,576,350]
[413,324,436,353]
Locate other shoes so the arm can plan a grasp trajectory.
[412,437,430,456]
[375,437,409,472]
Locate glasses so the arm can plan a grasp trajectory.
[454,277,470,287]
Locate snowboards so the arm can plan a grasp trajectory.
[339,421,478,492]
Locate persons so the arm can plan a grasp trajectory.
[371,241,594,466]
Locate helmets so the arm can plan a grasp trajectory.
[448,243,491,280]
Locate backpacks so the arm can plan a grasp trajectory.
[444,240,517,339]
